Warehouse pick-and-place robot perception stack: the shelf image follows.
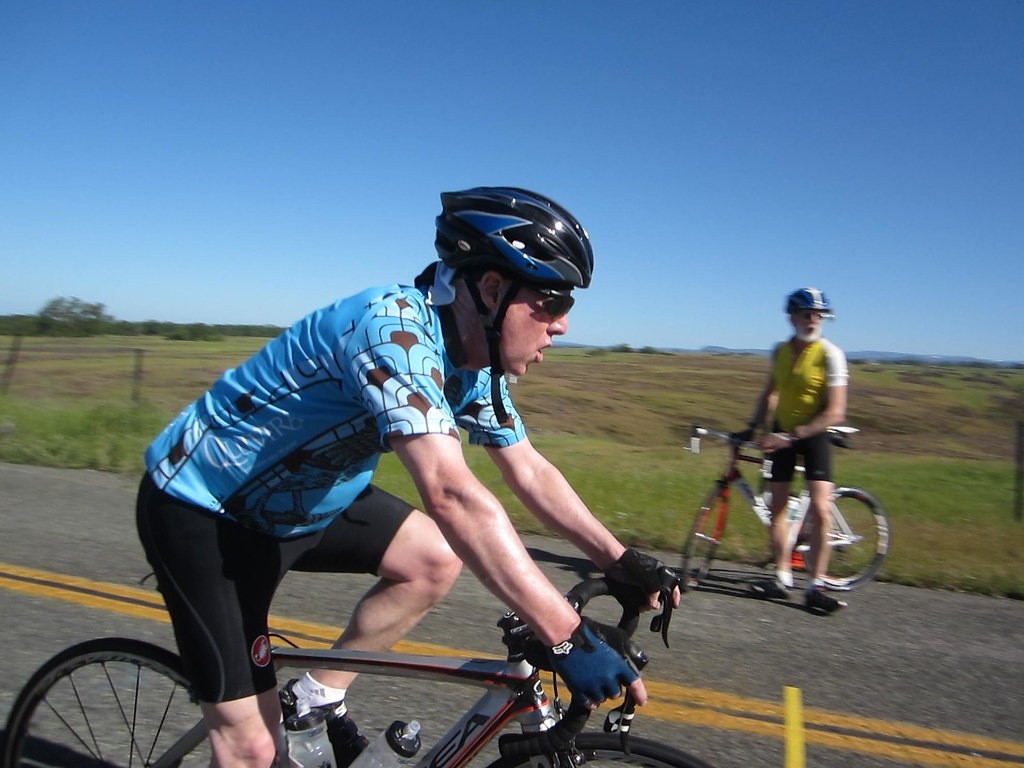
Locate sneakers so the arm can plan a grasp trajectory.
[749,574,792,593]
[277,678,370,768]
[805,585,839,612]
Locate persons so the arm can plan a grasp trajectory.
[131,183,683,768]
[727,283,843,613]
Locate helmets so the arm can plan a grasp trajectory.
[787,287,830,314]
[433,185,594,289]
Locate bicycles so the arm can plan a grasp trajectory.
[2,567,715,766]
[677,421,890,590]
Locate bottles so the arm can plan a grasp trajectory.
[347,719,422,768]
[285,698,336,768]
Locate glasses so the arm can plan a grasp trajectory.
[533,287,575,318]
